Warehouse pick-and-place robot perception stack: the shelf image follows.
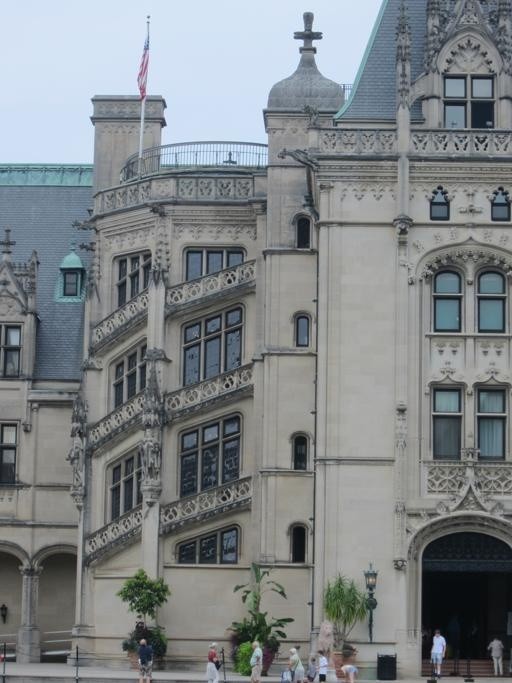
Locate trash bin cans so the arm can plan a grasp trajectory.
[377,653,397,680]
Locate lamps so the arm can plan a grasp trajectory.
[0,604,8,623]
[364,563,379,641]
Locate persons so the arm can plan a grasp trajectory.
[306,657,319,683]
[249,639,263,682]
[137,637,154,682]
[206,641,222,683]
[286,647,306,682]
[317,649,330,683]
[486,633,504,677]
[430,629,446,678]
[341,663,360,682]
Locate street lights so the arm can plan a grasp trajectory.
[362,562,380,642]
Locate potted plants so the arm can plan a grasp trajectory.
[223,563,295,675]
[316,570,370,679]
[114,569,171,671]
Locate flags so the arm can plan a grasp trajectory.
[137,12,153,102]
